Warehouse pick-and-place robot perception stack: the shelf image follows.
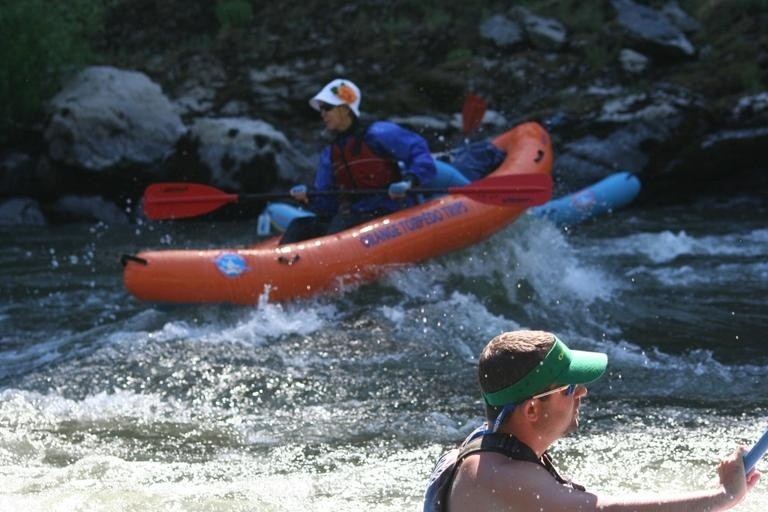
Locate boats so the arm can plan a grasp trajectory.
[260,169,640,238]
[118,121,550,303]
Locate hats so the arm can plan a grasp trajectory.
[481,333,608,406]
[308,79,361,118]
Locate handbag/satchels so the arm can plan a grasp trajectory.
[453,141,505,181]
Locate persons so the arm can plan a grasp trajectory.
[277,78,438,246]
[420,329,761,511]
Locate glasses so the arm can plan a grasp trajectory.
[320,104,335,111]
[533,384,577,399]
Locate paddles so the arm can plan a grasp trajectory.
[145,174,552,219]
[431,107,555,158]
[459,94,487,142]
